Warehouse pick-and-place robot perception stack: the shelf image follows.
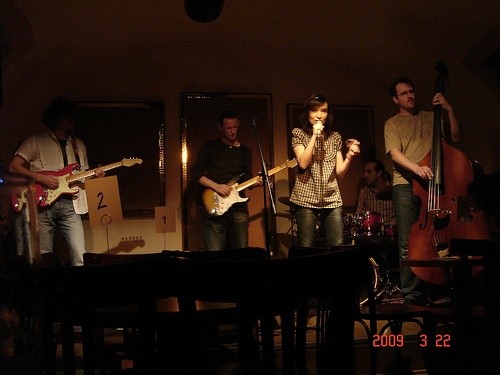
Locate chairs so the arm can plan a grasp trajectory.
[0,236,499,375]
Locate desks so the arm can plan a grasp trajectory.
[399,257,494,375]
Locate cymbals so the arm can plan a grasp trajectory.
[278,196,299,209]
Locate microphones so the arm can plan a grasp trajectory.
[250,111,256,123]
[317,120,323,137]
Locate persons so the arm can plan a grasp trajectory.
[288,95,361,248]
[4,142,29,256]
[354,160,396,241]
[383,76,462,304]
[193,110,263,251]
[10,96,104,267]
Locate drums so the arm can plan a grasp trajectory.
[358,256,384,306]
[350,210,384,243]
[314,212,353,242]
[378,225,401,246]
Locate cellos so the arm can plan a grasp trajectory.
[406,58,494,289]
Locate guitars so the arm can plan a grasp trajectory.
[10,155,146,214]
[202,158,298,218]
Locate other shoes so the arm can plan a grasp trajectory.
[423,297,434,307]
[403,298,417,306]
